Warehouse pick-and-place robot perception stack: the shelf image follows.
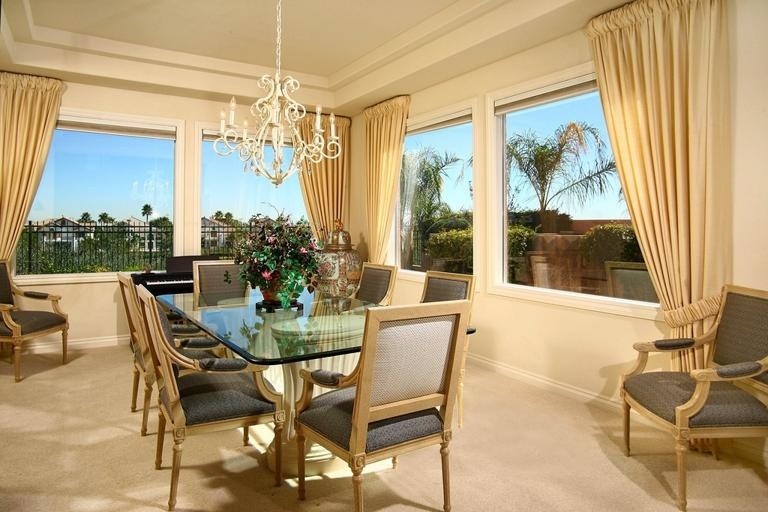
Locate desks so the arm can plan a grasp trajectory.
[156,293,390,478]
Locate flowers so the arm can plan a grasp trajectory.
[224,212,334,311]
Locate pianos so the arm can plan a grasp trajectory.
[131,272,194,296]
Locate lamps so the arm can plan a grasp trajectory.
[214,0,342,185]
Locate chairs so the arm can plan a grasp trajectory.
[192,291,250,311]
[193,260,250,293]
[344,262,398,308]
[136,284,286,512]
[419,271,476,428]
[294,298,475,512]
[117,271,235,437]
[618,284,768,511]
[0,258,69,383]
